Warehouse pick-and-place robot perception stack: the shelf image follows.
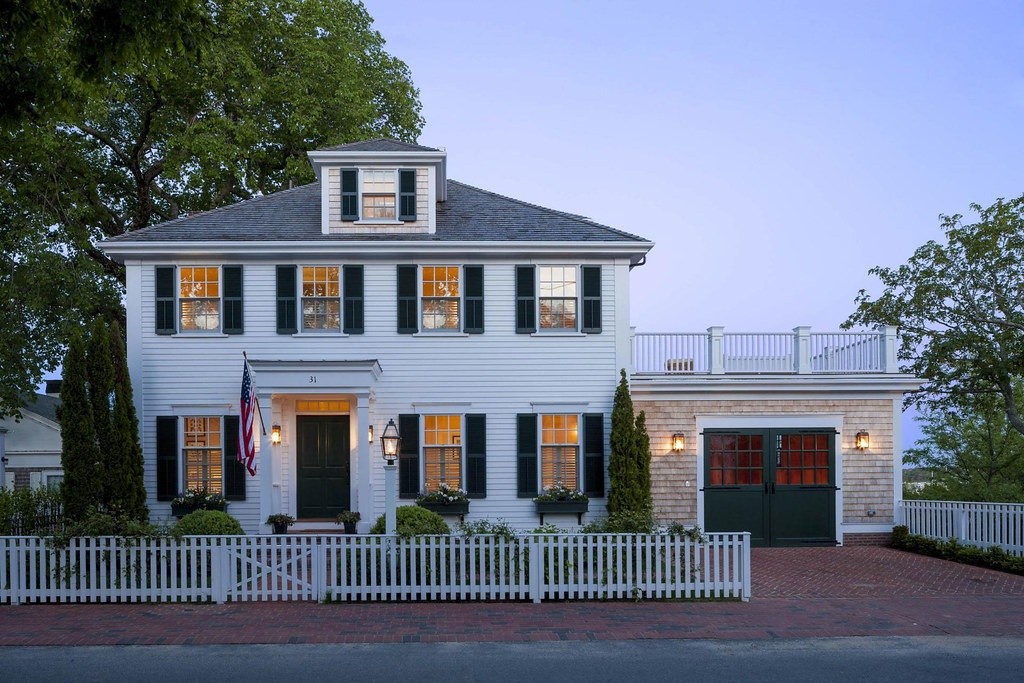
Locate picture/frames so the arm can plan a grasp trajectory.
[187,441,205,447]
[186,417,205,437]
[452,436,460,460]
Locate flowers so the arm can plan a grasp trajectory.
[534,480,588,502]
[414,482,472,503]
[170,486,226,505]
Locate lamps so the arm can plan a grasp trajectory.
[672,430,684,454]
[856,429,869,450]
[379,419,402,465]
[369,425,373,443]
[272,425,281,445]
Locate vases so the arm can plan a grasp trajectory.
[534,500,588,514]
[171,503,228,517]
[416,501,470,515]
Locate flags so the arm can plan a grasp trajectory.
[237,359,256,476]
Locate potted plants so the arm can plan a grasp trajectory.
[265,513,295,534]
[334,511,361,534]
